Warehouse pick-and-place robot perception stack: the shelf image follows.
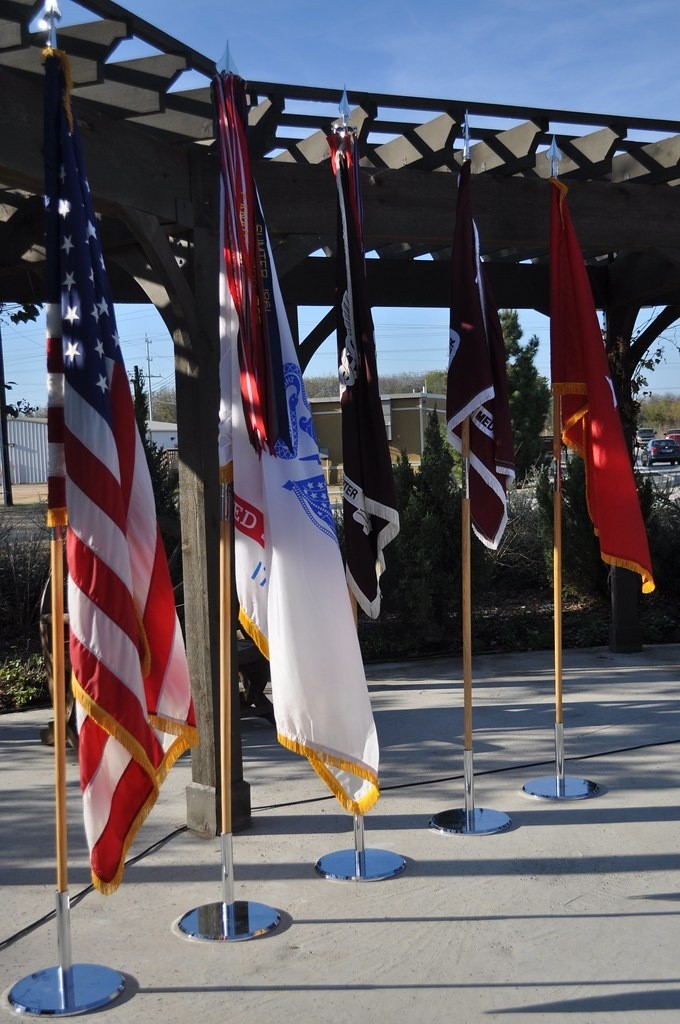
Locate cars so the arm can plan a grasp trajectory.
[663,429,680,445]
[640,439,680,467]
[550,451,574,470]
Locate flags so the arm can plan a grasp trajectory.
[445,160,517,551]
[209,76,380,815]
[330,130,400,619]
[554,181,656,594]
[40,55,198,891]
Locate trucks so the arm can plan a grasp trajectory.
[636,427,657,448]
